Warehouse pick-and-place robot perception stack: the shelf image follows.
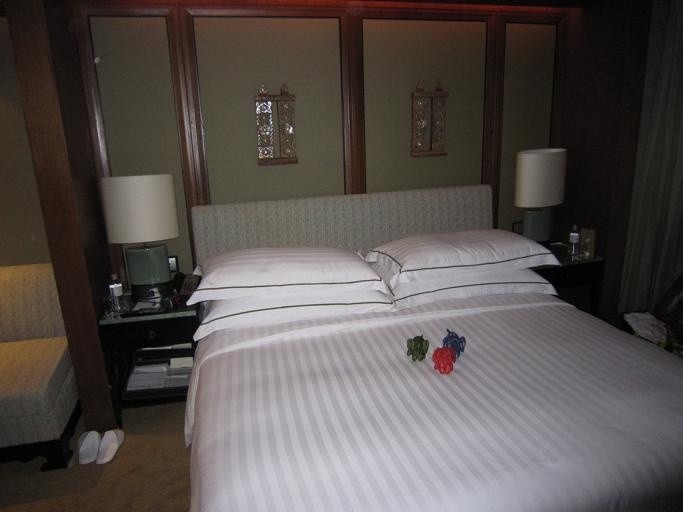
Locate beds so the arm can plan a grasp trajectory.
[184,185,683,512]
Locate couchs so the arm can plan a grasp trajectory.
[0,263,82,468]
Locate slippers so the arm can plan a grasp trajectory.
[77,430,102,466]
[95,428,126,465]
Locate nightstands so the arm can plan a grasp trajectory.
[531,243,605,318]
[99,295,200,430]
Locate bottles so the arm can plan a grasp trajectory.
[109,273,122,313]
[569,224,580,255]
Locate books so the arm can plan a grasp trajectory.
[123,339,193,392]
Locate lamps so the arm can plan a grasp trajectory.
[98,174,180,304]
[514,149,568,246]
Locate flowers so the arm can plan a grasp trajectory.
[404,328,465,374]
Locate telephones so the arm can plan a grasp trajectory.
[171,272,201,298]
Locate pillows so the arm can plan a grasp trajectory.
[356,230,563,289]
[185,247,391,307]
[191,291,398,341]
[383,269,558,314]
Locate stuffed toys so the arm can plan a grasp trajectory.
[405,333,429,363]
[432,347,456,375]
[442,328,466,358]
